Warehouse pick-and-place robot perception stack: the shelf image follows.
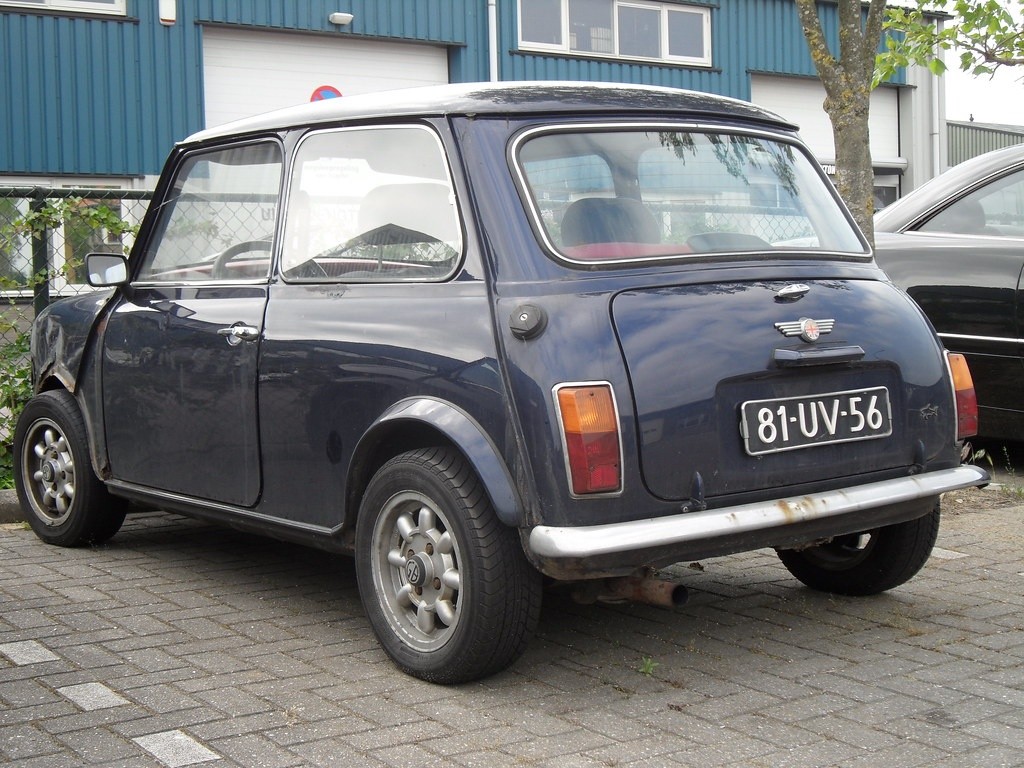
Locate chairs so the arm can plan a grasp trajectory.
[560,197,662,246]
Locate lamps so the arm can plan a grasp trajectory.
[328,12,354,24]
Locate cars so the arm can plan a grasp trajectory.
[759,141,1023,445]
[11,79,983,686]
